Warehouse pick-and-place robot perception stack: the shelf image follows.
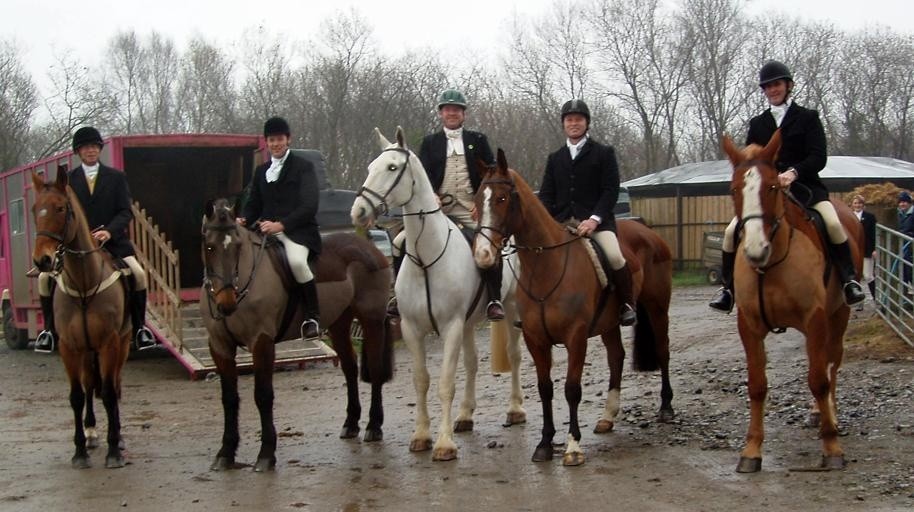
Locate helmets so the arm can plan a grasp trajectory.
[73,127,103,152]
[438,90,466,110]
[264,116,291,137]
[759,61,793,88]
[561,99,591,122]
[898,194,911,202]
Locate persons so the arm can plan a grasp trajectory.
[708,59,865,314]
[513,99,638,330]
[386,91,504,321]
[851,194,876,300]
[38,129,157,351]
[895,193,914,305]
[235,116,326,339]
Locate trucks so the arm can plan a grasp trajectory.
[0,135,338,379]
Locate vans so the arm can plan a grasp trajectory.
[314,190,395,286]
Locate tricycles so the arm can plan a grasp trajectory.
[704,232,724,284]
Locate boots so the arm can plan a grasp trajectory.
[612,262,636,326]
[514,317,523,327]
[36,295,57,349]
[832,240,867,307]
[487,267,506,320]
[387,298,398,315]
[707,250,735,314]
[299,278,320,338]
[131,289,155,350]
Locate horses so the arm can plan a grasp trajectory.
[721,130,865,474]
[473,147,674,467]
[350,125,526,462]
[200,199,394,474]
[31,162,133,470]
